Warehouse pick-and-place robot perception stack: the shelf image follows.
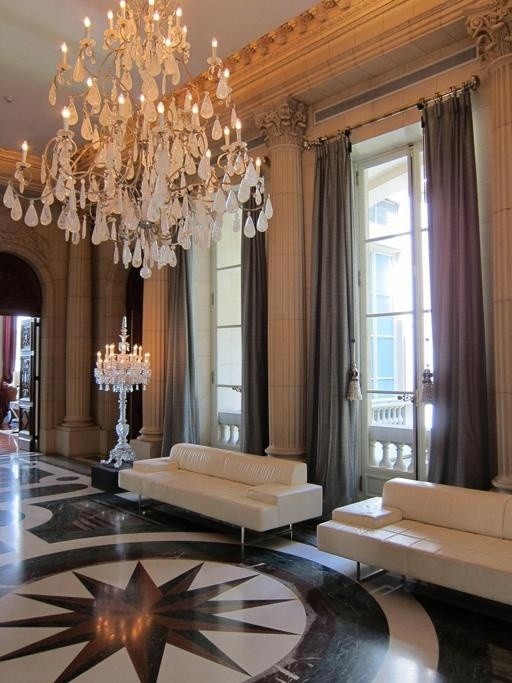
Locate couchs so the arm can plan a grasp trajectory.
[118,443,323,544]
[317,478,511,608]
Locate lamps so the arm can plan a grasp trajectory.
[2,1,274,280]
[93,314,151,468]
